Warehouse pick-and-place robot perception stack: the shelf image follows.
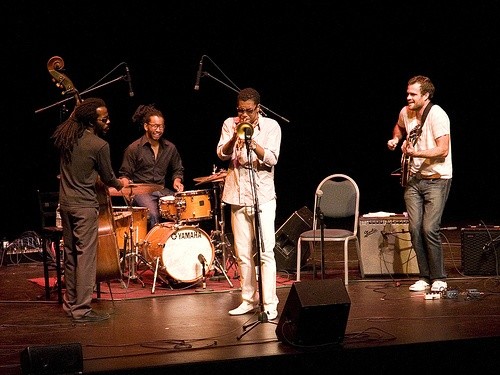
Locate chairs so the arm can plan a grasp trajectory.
[296,173,364,286]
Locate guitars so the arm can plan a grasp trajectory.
[400,123,422,187]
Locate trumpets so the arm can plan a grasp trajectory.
[236,116,259,151]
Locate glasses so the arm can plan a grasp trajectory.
[98,116,109,123]
[236,104,257,114]
[148,124,165,130]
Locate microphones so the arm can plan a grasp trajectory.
[381,231,388,240]
[198,254,208,267]
[194,58,203,91]
[482,244,490,252]
[244,128,251,144]
[126,65,134,96]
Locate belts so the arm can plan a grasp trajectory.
[415,171,440,179]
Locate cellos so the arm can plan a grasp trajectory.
[46,55,123,307]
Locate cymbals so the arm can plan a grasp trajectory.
[195,171,228,186]
[108,184,164,197]
[193,175,223,182]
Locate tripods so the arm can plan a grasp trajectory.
[120,187,174,290]
[209,182,239,278]
[237,144,278,340]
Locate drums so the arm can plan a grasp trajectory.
[143,222,215,284]
[158,195,177,218]
[111,206,150,251]
[174,189,213,222]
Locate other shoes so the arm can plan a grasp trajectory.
[431,280,448,291]
[72,311,110,322]
[408,280,431,291]
[228,299,259,315]
[263,303,278,320]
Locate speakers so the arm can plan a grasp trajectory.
[276,278,352,349]
[359,214,420,275]
[274,206,326,271]
[461,227,500,275]
[21,343,83,375]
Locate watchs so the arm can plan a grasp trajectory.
[250,141,257,150]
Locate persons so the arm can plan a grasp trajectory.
[387,76,452,290]
[119,105,184,229]
[52,98,129,322]
[216,87,281,320]
